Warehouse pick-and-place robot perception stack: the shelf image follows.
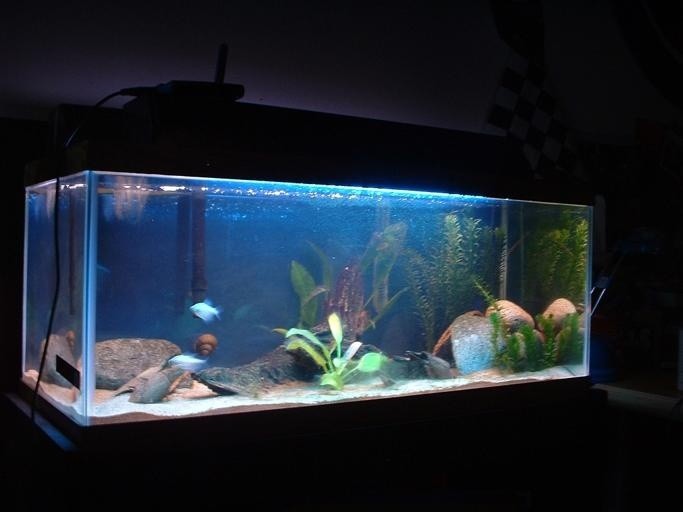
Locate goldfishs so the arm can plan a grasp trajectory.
[189,298,221,324]
[167,351,207,373]
